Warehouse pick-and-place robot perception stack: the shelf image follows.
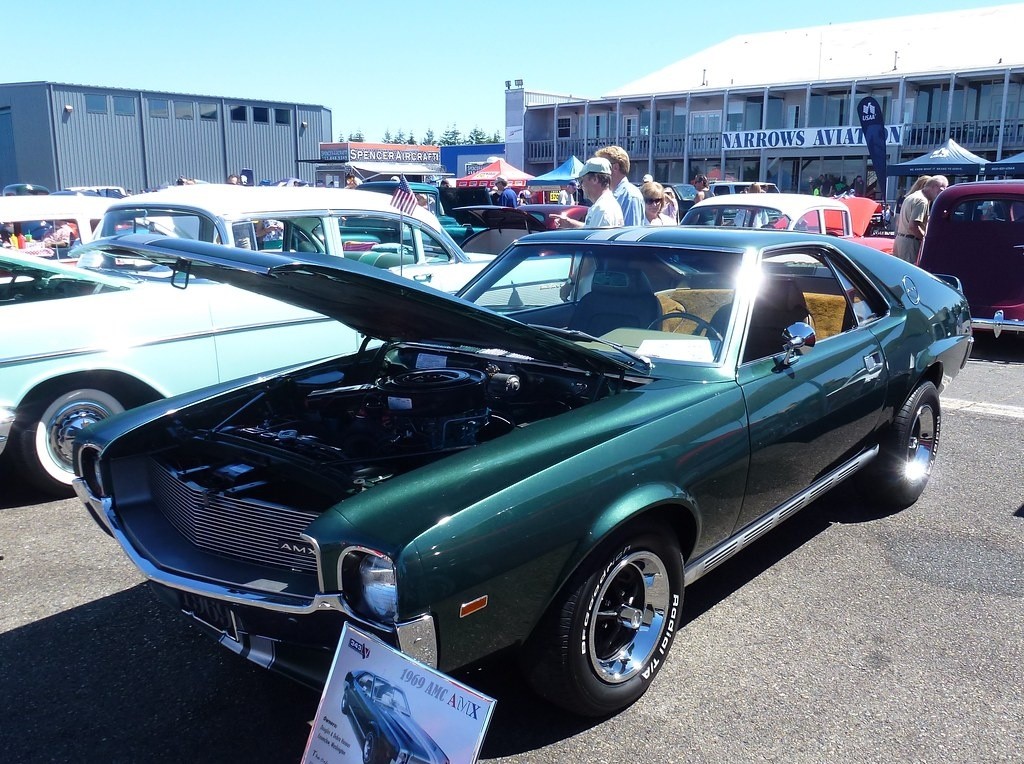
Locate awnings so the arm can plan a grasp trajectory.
[349,163,431,173]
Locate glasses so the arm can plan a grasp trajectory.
[695,181,701,184]
[645,198,661,205]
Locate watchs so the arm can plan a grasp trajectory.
[566,278,574,286]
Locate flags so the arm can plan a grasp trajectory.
[391,175,417,216]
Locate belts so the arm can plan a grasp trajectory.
[897,234,920,241]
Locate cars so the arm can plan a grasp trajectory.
[65,225,974,718]
[0,185,505,496]
[461,206,669,226]
[670,192,895,256]
[917,181,1024,332]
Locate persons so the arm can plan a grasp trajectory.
[487,145,771,305]
[0,174,363,250]
[806,174,1024,266]
[391,176,451,215]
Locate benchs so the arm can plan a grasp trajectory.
[343,250,448,270]
[670,288,863,342]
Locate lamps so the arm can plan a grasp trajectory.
[515,80,523,88]
[505,81,511,89]
[302,122,307,127]
[64,105,73,112]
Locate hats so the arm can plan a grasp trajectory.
[579,157,613,178]
[569,179,579,188]
[643,174,653,182]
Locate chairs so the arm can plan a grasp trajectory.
[705,281,814,364]
[568,267,663,339]
[871,217,892,238]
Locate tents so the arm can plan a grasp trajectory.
[456,156,587,204]
[886,137,1024,213]
